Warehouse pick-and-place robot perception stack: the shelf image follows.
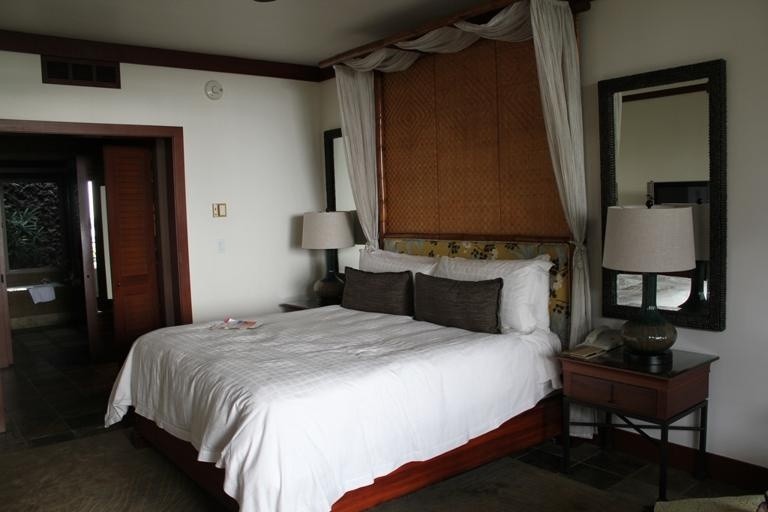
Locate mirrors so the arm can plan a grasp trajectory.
[598,59,725,331]
[323,129,367,280]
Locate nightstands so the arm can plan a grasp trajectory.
[558,349,719,501]
[278,300,317,312]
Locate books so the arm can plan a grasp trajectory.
[569,345,606,360]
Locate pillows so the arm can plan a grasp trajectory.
[533,253,554,333]
[433,254,556,334]
[413,272,503,333]
[359,248,433,282]
[342,266,412,316]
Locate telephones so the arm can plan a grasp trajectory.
[581,326,623,351]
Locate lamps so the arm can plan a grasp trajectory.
[302,212,355,307]
[602,201,696,355]
[662,203,710,309]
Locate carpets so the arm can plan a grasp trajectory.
[0,427,649,512]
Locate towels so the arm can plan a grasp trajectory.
[27,284,57,304]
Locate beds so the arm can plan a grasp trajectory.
[616,272,707,311]
[101,234,573,512]
